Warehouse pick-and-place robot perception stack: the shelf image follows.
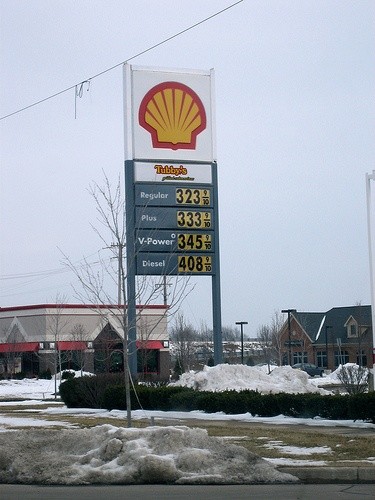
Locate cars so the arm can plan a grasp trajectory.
[291,362,325,377]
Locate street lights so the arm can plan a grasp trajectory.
[281,308,297,366]
[325,325,333,370]
[235,321,248,364]
